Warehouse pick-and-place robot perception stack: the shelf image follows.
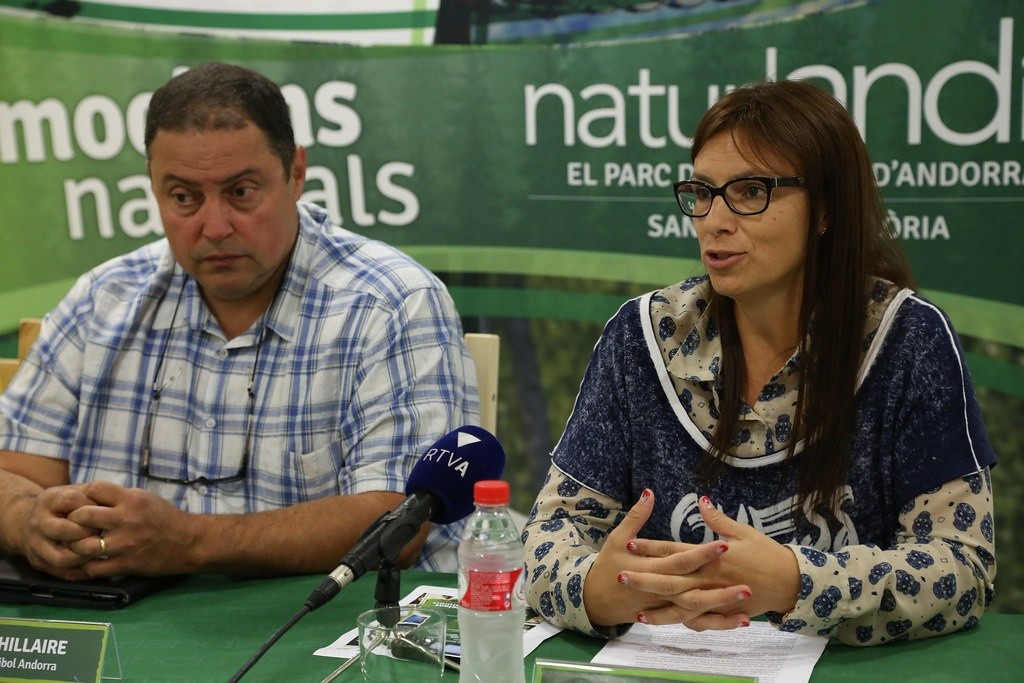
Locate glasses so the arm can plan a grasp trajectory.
[140,380,256,485]
[672,176,805,219]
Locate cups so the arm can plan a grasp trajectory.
[357,605,446,683]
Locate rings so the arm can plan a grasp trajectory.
[100,535,107,556]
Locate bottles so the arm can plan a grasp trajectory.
[456,481,528,683]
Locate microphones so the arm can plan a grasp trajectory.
[305,426,505,610]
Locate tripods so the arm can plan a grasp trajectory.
[323,510,463,683]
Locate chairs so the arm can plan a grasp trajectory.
[11,319,506,442]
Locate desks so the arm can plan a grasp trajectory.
[0,565,1023,683]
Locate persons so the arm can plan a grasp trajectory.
[1,64,481,580]
[523,82,996,646]
